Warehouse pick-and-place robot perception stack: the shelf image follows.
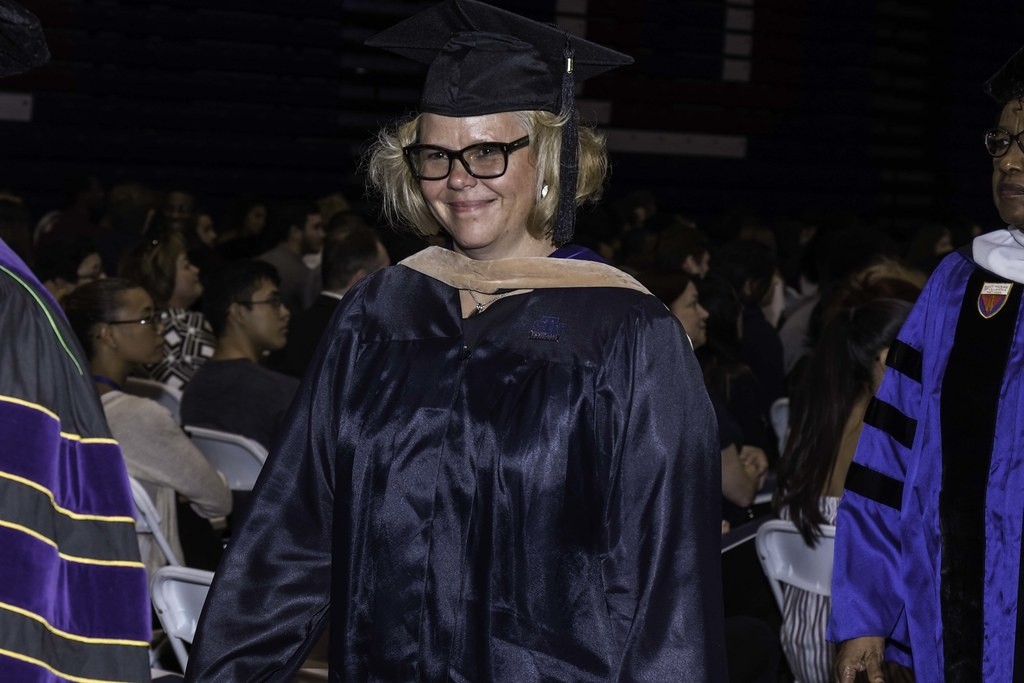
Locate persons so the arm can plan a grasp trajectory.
[1,0,1024,683]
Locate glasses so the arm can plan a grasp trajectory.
[96,307,172,336]
[400,135,529,180]
[985,125,1024,160]
[242,291,291,310]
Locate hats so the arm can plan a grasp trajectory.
[363,1,631,246]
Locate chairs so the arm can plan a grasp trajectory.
[717,396,838,619]
[124,376,271,672]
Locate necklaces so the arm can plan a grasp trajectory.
[92,375,120,390]
